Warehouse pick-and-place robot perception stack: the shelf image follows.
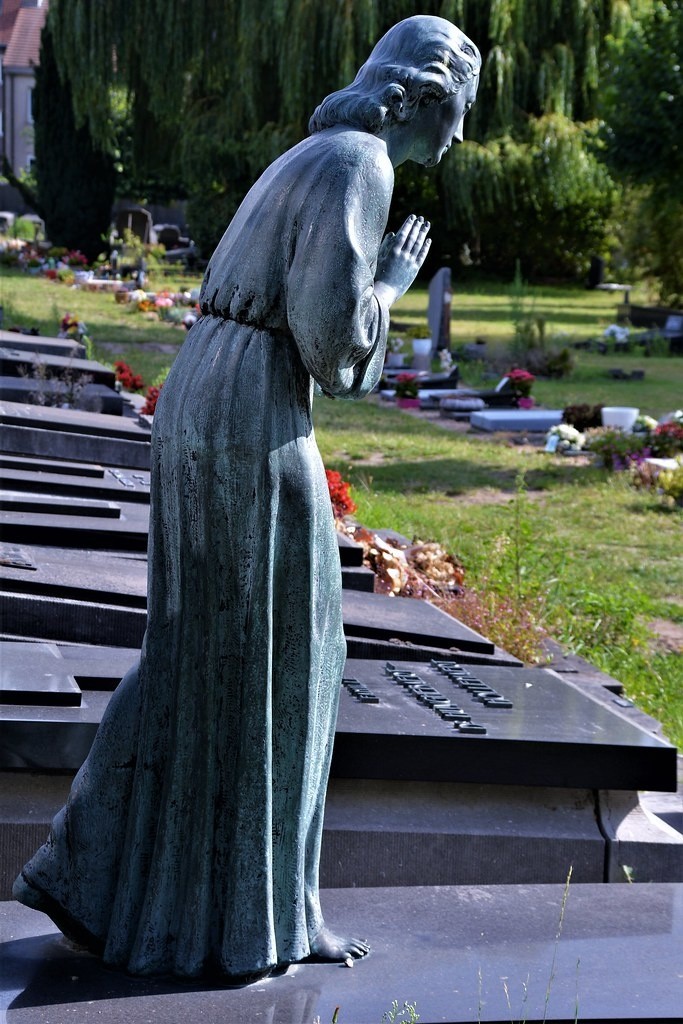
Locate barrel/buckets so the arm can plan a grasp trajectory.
[413,338,431,352]
[602,406,639,433]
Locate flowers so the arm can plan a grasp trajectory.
[60,312,93,350]
[395,372,421,398]
[439,349,456,375]
[114,361,144,392]
[506,371,537,399]
[128,288,206,331]
[141,384,165,417]
[546,405,683,471]
[326,468,358,530]
[20,244,108,282]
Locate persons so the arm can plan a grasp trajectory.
[12,14,482,984]
[604,314,630,343]
